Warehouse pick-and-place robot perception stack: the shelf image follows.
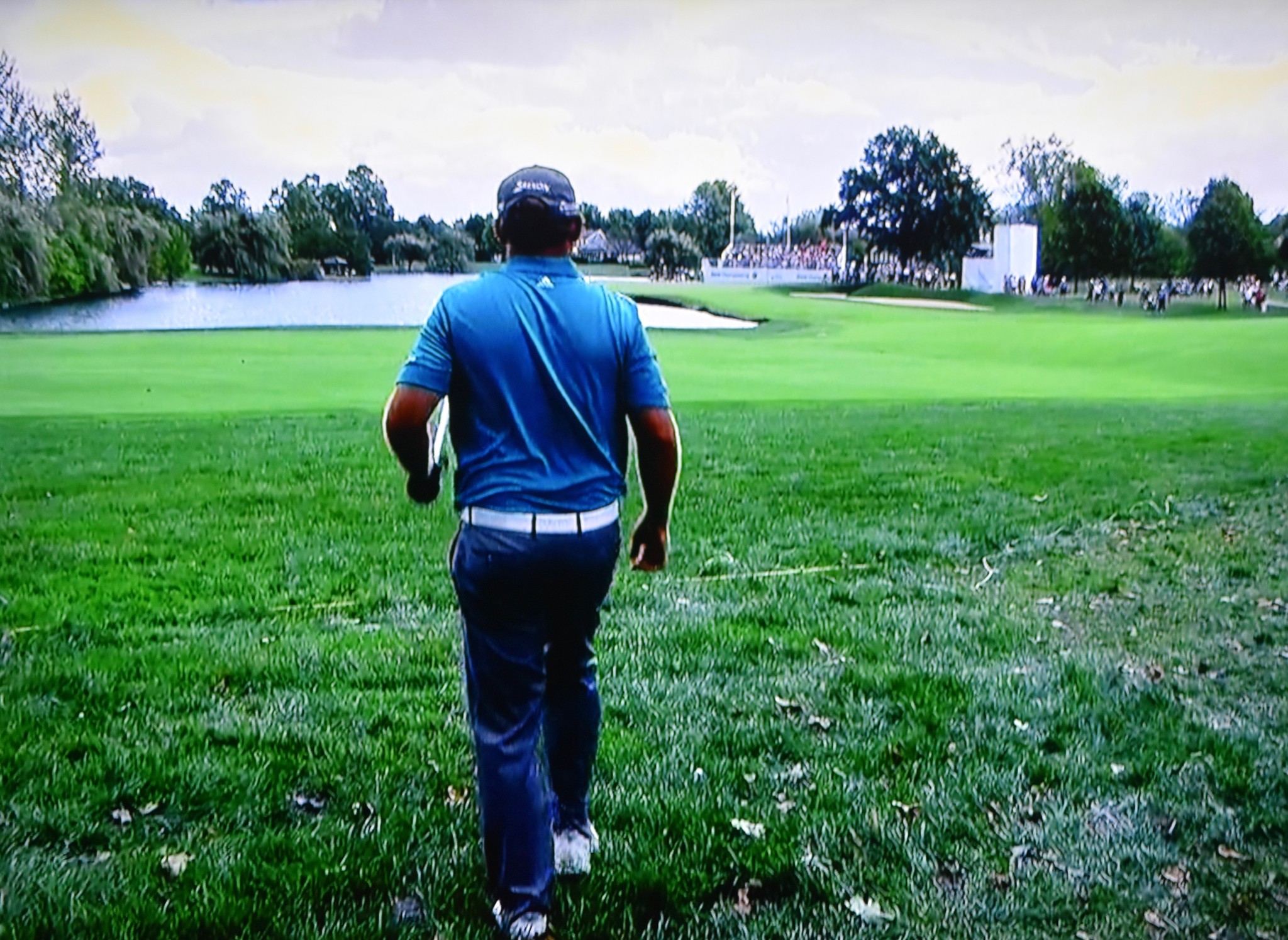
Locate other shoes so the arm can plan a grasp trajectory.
[548,815,599,876]
[493,898,547,940]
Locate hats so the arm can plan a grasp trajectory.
[496,166,578,218]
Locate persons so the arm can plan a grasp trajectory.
[1087,278,1128,310]
[1136,275,1214,313]
[380,166,680,939]
[874,264,959,291]
[1237,272,1268,315]
[1004,273,1067,299]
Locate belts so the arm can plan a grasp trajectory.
[460,499,620,534]
